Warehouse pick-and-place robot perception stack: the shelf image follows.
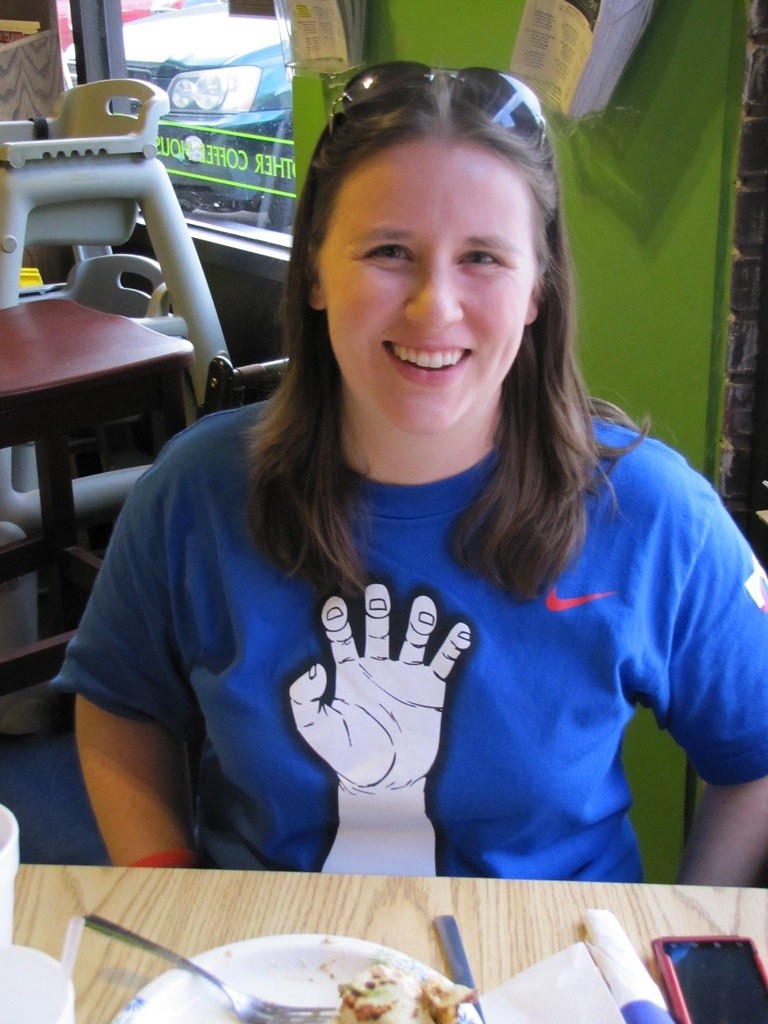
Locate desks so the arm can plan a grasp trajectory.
[12,862,768,1024]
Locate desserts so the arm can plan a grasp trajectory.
[335,963,477,1024]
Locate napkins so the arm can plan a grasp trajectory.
[469,940,628,1024]
[581,904,668,1012]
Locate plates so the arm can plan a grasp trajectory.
[108,934,482,1024]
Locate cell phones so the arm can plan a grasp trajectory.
[653,934,768,1024]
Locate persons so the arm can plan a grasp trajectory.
[50,61,768,888]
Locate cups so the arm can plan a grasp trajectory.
[0,944,77,1024]
[0,803,22,946]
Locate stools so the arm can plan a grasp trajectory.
[0,298,196,727]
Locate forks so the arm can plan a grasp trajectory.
[84,916,340,1024]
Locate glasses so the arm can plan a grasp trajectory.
[328,61,548,150]
